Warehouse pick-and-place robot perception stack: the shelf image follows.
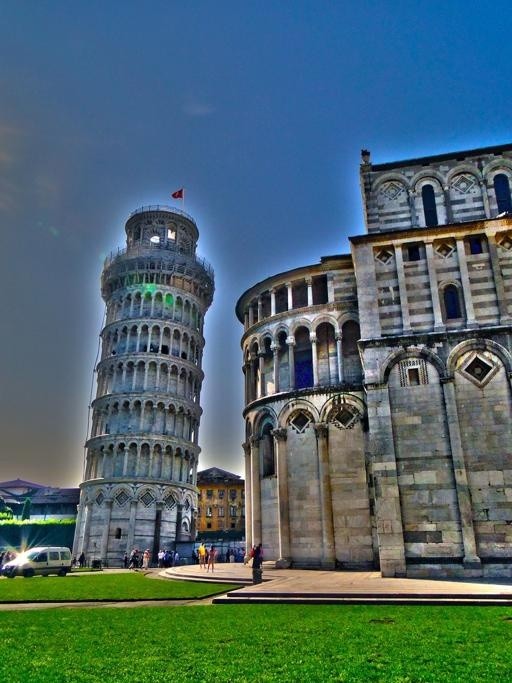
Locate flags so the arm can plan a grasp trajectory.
[170,186,184,199]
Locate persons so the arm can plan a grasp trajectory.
[1,548,18,577]
[78,551,85,567]
[124,551,129,568]
[191,549,198,564]
[127,547,180,572]
[224,546,246,562]
[196,538,208,570]
[206,544,219,574]
[249,542,265,586]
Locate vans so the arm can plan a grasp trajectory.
[2,544,75,578]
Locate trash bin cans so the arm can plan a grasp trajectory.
[92,560,102,568]
[253,569,263,585]
